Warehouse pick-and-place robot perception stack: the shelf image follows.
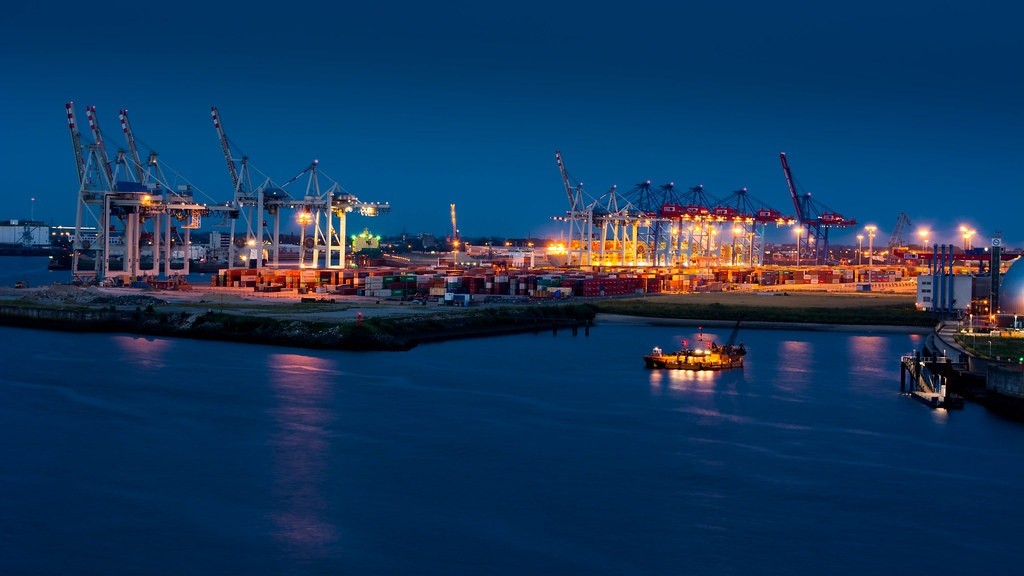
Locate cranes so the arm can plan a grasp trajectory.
[550,148,858,268]
[65,101,392,275]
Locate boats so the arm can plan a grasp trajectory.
[48,232,267,272]
[641,332,746,369]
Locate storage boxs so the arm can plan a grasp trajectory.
[210,261,1007,306]
